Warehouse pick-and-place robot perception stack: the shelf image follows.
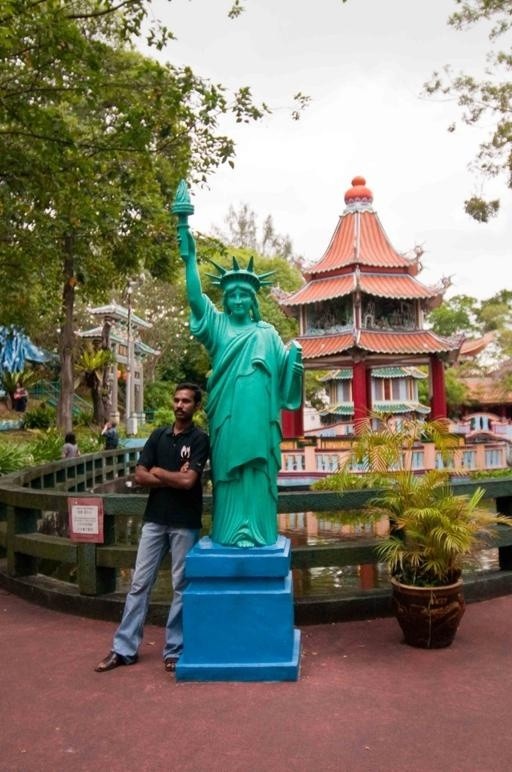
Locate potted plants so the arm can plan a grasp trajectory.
[307,405,511,649]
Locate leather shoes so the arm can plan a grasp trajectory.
[164,657,179,672]
[94,652,132,672]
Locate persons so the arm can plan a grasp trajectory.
[100,419,120,452]
[12,381,30,412]
[169,178,306,550]
[60,432,82,460]
[91,379,212,674]
